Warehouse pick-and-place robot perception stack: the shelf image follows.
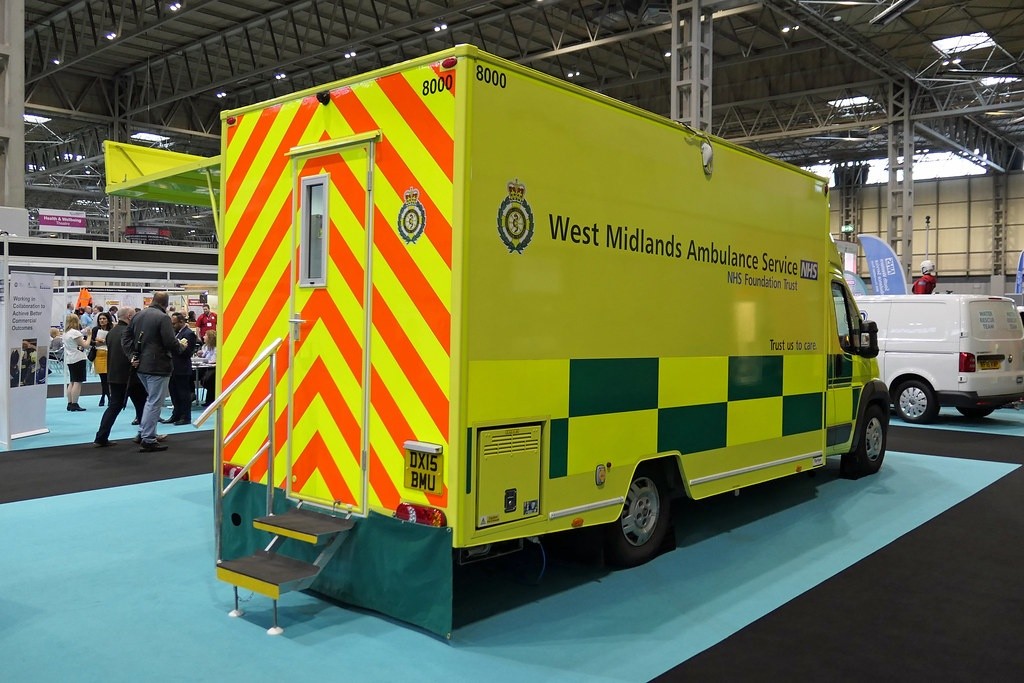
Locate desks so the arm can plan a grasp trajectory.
[191,363,216,409]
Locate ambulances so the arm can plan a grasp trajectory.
[102,44,892,637]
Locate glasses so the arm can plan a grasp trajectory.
[172,321,180,326]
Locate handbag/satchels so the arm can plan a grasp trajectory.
[87,346,98,362]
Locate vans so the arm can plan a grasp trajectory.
[834,294,1024,424]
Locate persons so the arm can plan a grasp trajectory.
[87,313,113,407]
[21,341,37,385]
[93,306,168,449]
[122,290,188,452]
[62,314,92,411]
[911,260,936,294]
[197,330,216,407]
[161,312,197,426]
[48,302,149,374]
[169,304,217,353]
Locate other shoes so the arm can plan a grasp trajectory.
[67,403,86,411]
[158,418,165,422]
[137,441,169,452]
[161,417,180,423]
[93,441,117,448]
[174,419,191,425]
[134,433,167,442]
[132,418,140,425]
[201,403,211,407]
[108,400,110,406]
[99,399,104,406]
